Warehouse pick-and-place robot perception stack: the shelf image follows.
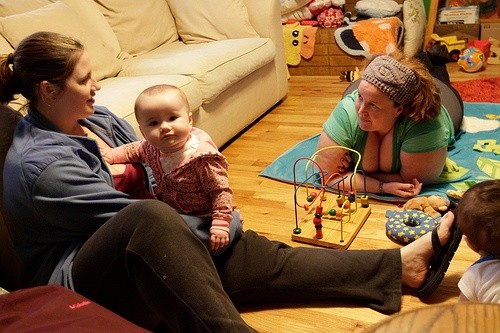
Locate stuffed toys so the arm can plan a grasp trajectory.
[402,194,458,219]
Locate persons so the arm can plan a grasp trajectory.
[317,42,465,198]
[98,84,232,247]
[453,179,500,305]
[0,31,463,333]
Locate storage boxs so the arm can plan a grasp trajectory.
[438,5,480,23]
[436,23,481,43]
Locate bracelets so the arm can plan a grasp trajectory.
[379,181,384,194]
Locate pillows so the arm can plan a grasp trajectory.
[0,0,131,80]
[95,0,178,57]
[169,0,255,44]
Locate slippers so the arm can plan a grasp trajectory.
[415,207,464,297]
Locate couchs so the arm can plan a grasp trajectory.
[0,0,291,149]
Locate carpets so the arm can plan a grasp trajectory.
[259,76,500,205]
